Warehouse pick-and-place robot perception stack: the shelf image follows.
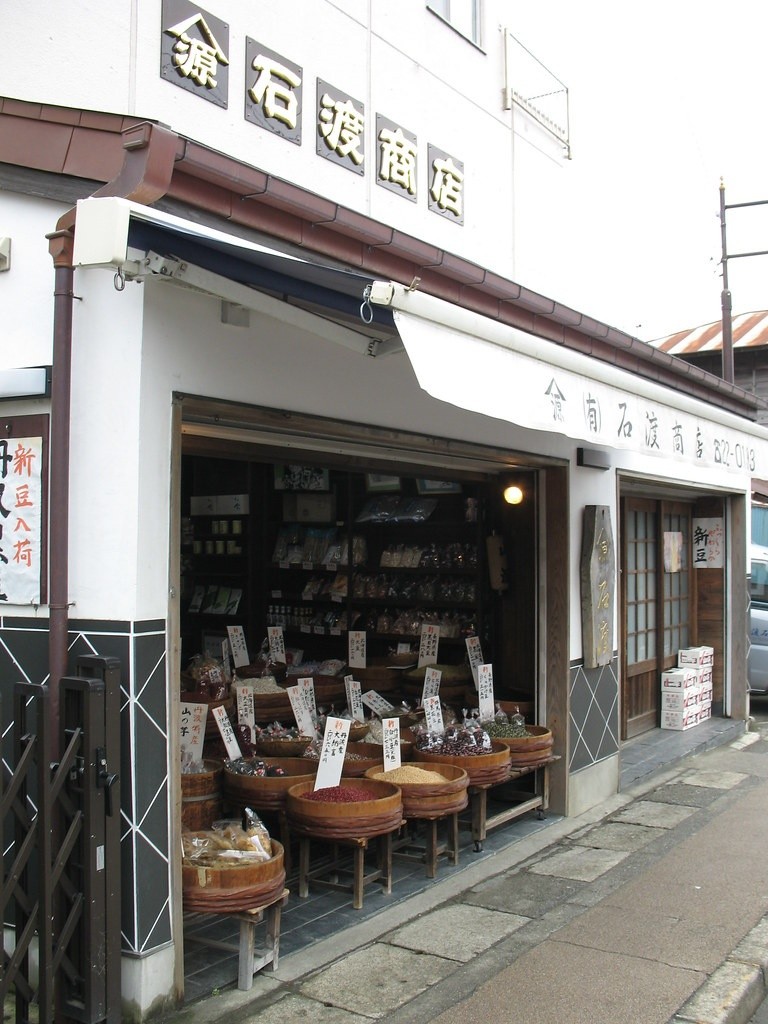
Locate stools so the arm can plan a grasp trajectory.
[183,889,290,991]
[299,819,407,909]
[379,812,458,878]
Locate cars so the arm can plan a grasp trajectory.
[747,542,767,706]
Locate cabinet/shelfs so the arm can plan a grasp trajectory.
[182,456,499,674]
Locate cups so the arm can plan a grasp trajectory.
[226,541,236,554]
[193,541,201,554]
[212,520,228,535]
[233,520,241,534]
[206,541,214,554]
[216,541,223,554]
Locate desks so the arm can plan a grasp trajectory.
[469,754,561,852]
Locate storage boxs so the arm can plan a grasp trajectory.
[181,653,559,912]
[660,646,714,731]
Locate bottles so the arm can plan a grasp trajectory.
[266,605,313,629]
